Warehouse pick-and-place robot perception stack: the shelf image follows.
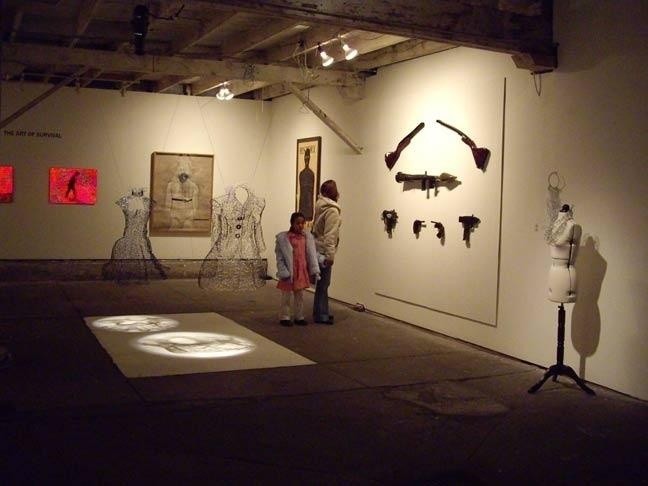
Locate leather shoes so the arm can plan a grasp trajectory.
[280,313,335,327]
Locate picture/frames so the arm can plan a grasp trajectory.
[295,135,322,231]
[149,151,215,237]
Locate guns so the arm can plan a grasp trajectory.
[385,122,425,169]
[459,215,479,240]
[396,171,461,199]
[414,220,425,233]
[437,120,489,169]
[431,221,444,238]
[383,209,398,233]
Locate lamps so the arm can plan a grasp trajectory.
[339,38,358,61]
[316,41,335,68]
[216,84,234,101]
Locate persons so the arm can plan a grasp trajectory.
[65,172,81,199]
[299,148,315,221]
[547,205,582,304]
[165,171,199,231]
[310,180,341,324]
[275,212,321,326]
